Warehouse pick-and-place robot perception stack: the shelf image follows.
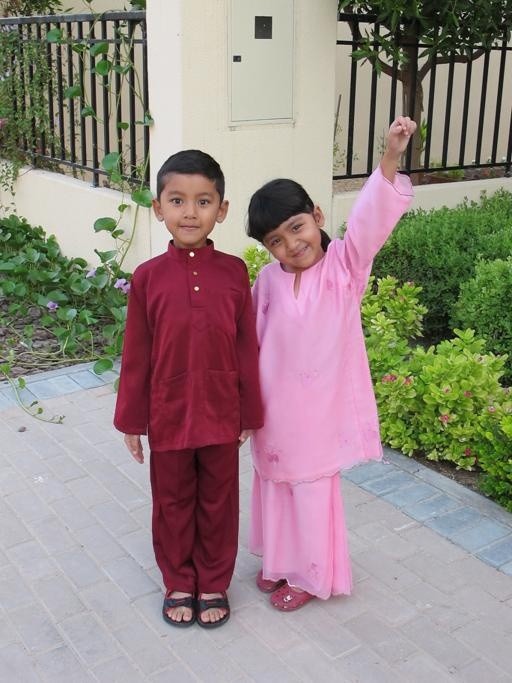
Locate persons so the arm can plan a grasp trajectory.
[244,114,418,613]
[113,149,263,629]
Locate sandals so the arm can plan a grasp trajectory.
[196,591,230,628]
[162,589,196,627]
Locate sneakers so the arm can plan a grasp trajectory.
[256,571,278,592]
[270,582,314,611]
